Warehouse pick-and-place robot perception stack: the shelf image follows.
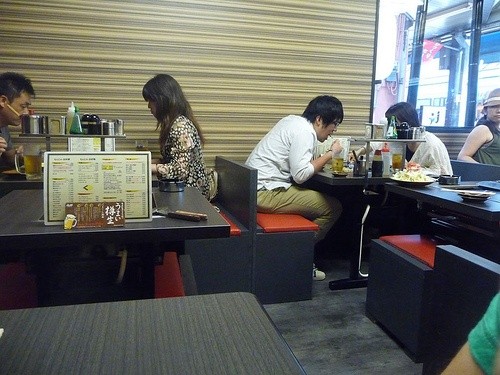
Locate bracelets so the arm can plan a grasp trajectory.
[329,150,332,151]
[362,146,366,153]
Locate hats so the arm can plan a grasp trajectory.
[483,88,500,107]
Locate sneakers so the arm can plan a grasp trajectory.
[312,264,326,280]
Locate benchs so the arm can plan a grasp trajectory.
[179,155,319,302]
[361,224,500,374]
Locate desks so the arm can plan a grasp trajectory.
[384,178,499,253]
[0,182,228,308]
[0,291,306,374]
[310,166,437,292]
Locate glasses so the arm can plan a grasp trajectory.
[332,120,339,127]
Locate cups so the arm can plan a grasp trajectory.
[19,110,40,134]
[51,113,60,134]
[115,119,124,136]
[135,140,148,151]
[391,149,403,171]
[332,139,345,174]
[354,160,366,176]
[15,144,42,181]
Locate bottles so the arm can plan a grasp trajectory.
[399,122,409,139]
[381,143,392,176]
[66,102,75,134]
[371,150,383,178]
[386,116,398,139]
[70,106,83,134]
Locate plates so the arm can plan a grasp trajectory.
[478,181,500,190]
[458,192,491,202]
[391,177,435,186]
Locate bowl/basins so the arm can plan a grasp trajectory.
[410,127,425,139]
[97,121,114,135]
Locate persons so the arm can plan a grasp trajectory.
[246,96,344,280]
[143,74,212,200]
[440,293,500,375]
[0,72,36,168]
[457,88,500,165]
[347,102,454,252]
